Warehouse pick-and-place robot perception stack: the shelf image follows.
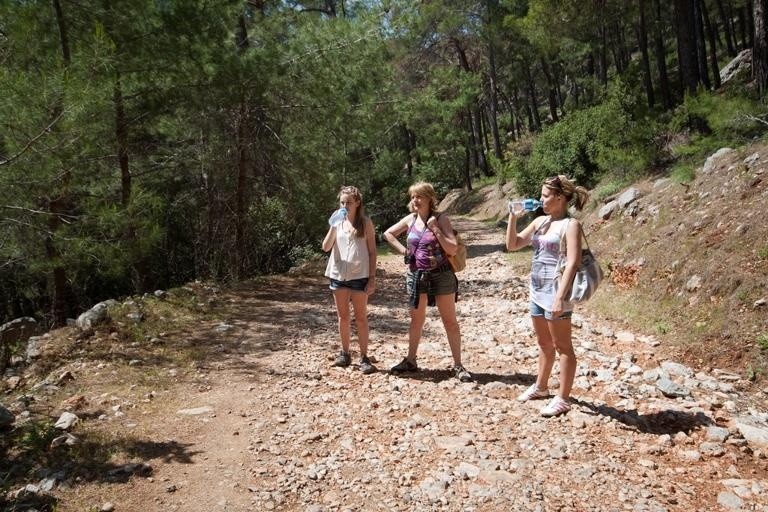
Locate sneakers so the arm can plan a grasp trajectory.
[389,355,420,375]
[330,351,353,368]
[539,395,573,418]
[357,356,379,376]
[451,364,473,383]
[515,381,552,404]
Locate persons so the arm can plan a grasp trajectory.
[382,182,473,381]
[503,174,604,418]
[322,185,379,375]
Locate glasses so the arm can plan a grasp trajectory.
[550,174,564,195]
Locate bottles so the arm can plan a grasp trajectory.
[509,199,543,212]
[328,207,348,226]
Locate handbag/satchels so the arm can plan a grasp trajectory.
[434,211,470,274]
[552,217,607,308]
